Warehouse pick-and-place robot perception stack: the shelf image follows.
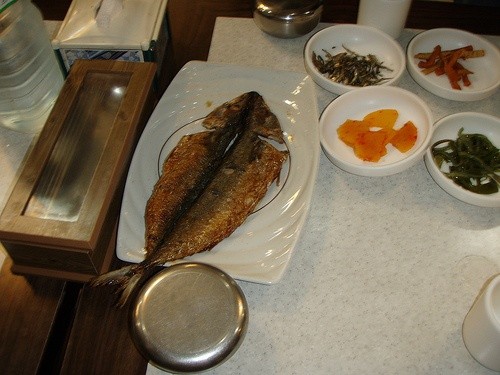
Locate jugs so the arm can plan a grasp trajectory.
[255,0,324,39]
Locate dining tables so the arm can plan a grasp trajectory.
[0,0,500,375]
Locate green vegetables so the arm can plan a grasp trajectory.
[431,128,500,195]
[311,43,394,87]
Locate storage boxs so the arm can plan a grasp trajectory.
[0,60,158,283]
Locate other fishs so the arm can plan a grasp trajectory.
[87,91,291,311]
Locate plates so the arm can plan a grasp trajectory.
[302,24,406,94]
[425,111,500,208]
[115,60,320,288]
[407,28,500,101]
[319,85,436,177]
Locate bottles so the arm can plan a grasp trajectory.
[357,0,412,39]
[462,274,500,372]
[131,262,251,374]
[0,0,65,131]
